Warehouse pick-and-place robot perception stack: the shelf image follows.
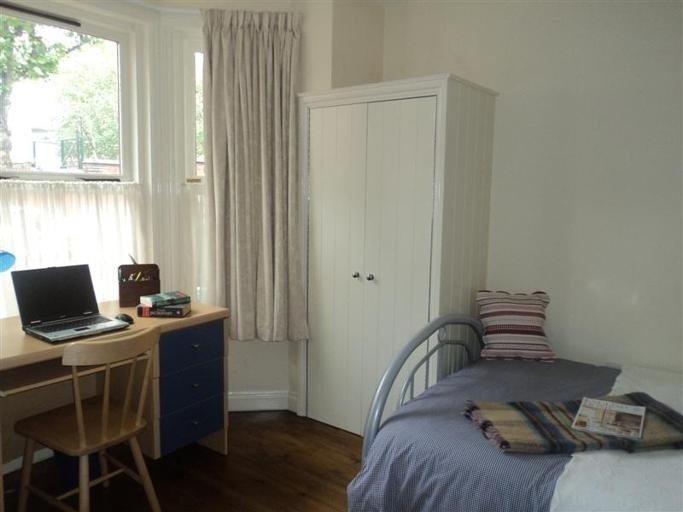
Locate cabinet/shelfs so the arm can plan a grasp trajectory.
[287,70,502,445]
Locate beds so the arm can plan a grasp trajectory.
[337,309,681,511]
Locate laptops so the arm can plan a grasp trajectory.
[10,263,130,344]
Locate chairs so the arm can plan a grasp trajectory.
[10,322,165,511]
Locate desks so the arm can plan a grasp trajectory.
[0,295,232,512]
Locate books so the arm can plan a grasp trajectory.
[140,290,190,308]
[136,303,191,318]
[572,396,646,440]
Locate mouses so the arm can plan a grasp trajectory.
[116,314,135,324]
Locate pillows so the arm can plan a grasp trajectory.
[468,287,557,364]
